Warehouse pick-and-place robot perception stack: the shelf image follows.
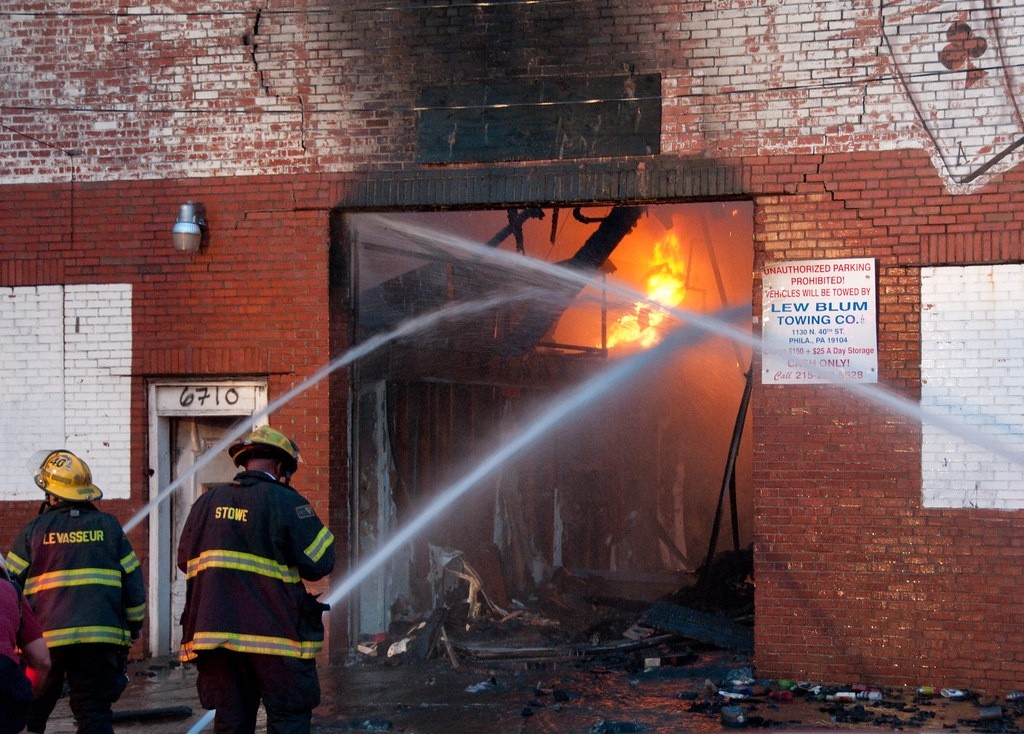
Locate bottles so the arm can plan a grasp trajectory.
[941,687,968,698]
[721,706,749,726]
[1006,690,1024,700]
[826,692,856,702]
[917,686,943,696]
[858,691,882,701]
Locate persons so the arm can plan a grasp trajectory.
[0,553,51,734]
[176,425,336,734]
[3,448,147,734]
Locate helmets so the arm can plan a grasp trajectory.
[228,427,300,477]
[35,450,103,502]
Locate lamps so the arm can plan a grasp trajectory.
[173,203,205,253]
